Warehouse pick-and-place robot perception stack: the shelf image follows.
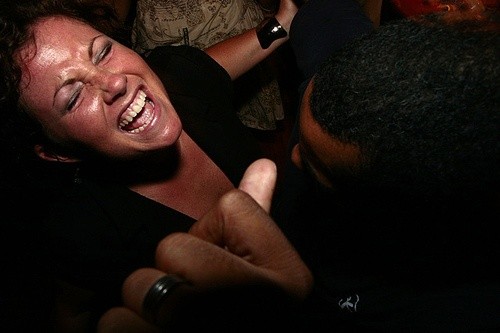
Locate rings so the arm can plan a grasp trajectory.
[140,275,184,328]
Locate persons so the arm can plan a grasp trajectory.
[3,1,314,333]
[95,1,500,333]
[84,2,285,185]
